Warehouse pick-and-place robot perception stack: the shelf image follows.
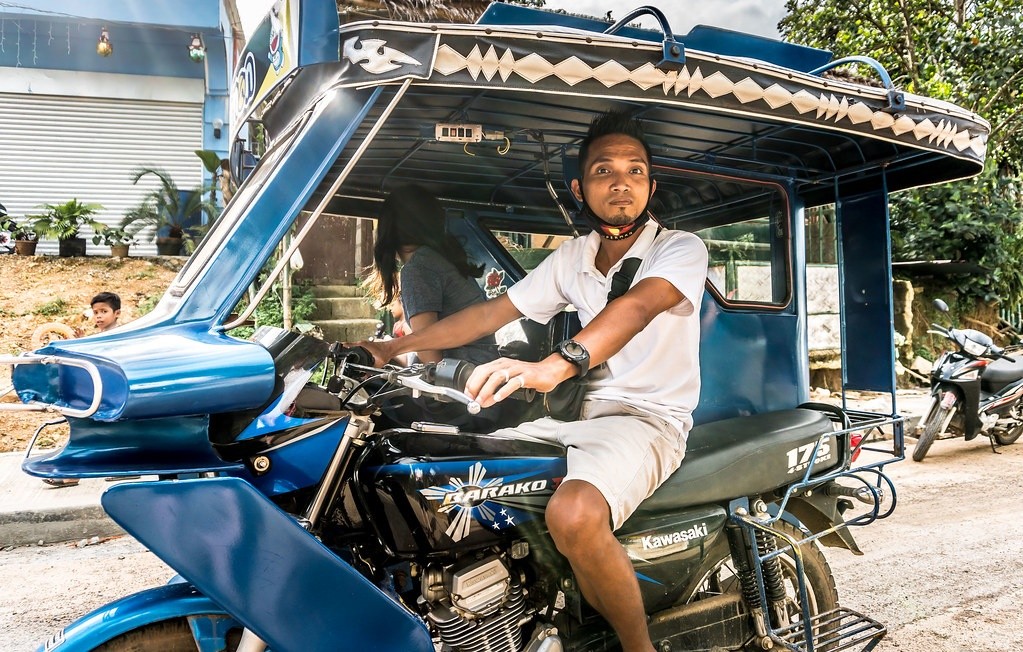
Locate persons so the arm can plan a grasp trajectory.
[388,290,412,338]
[41,292,141,487]
[372,183,500,367]
[341,111,708,652]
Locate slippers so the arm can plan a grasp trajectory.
[104,476,141,482]
[41,478,79,487]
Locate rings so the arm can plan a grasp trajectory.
[501,370,509,382]
[516,376,524,388]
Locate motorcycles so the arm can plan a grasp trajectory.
[911,298,1023,460]
[8,0,995,652]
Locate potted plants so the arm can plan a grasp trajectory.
[92,224,138,256]
[193,150,223,247]
[121,166,220,256]
[25,198,108,257]
[0,216,52,256]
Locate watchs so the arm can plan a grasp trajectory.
[550,339,590,379]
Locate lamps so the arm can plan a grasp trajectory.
[188,32,205,63]
[94,22,113,57]
[210,117,224,138]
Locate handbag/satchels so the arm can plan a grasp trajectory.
[543,375,590,421]
